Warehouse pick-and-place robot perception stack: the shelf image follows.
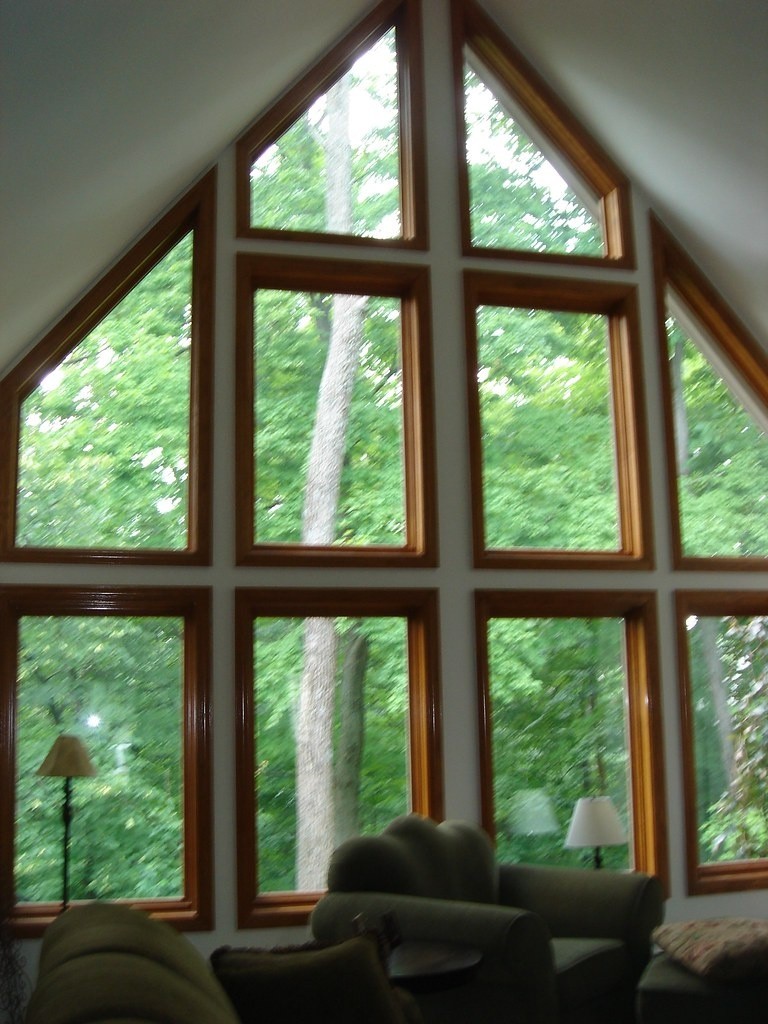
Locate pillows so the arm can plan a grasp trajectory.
[649,916,768,985]
[210,922,411,1024]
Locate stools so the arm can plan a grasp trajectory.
[637,949,768,1024]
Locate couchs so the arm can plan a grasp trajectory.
[305,815,669,1024]
[25,903,429,1024]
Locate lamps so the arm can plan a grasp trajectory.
[32,734,100,919]
[564,795,630,872]
